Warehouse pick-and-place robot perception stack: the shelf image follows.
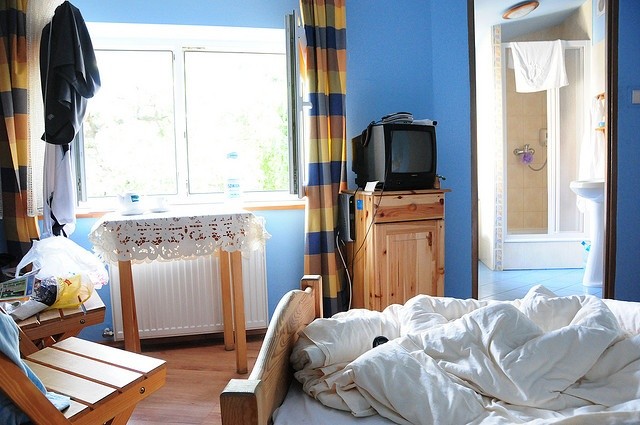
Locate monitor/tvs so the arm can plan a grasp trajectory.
[352,122,437,192]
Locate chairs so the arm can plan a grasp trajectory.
[0,333,167,424]
[0,274,105,355]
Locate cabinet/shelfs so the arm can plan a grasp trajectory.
[345,190,445,312]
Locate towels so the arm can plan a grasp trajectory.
[507,40,570,94]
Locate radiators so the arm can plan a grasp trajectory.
[109,218,269,341]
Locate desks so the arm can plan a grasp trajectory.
[104,204,252,375]
